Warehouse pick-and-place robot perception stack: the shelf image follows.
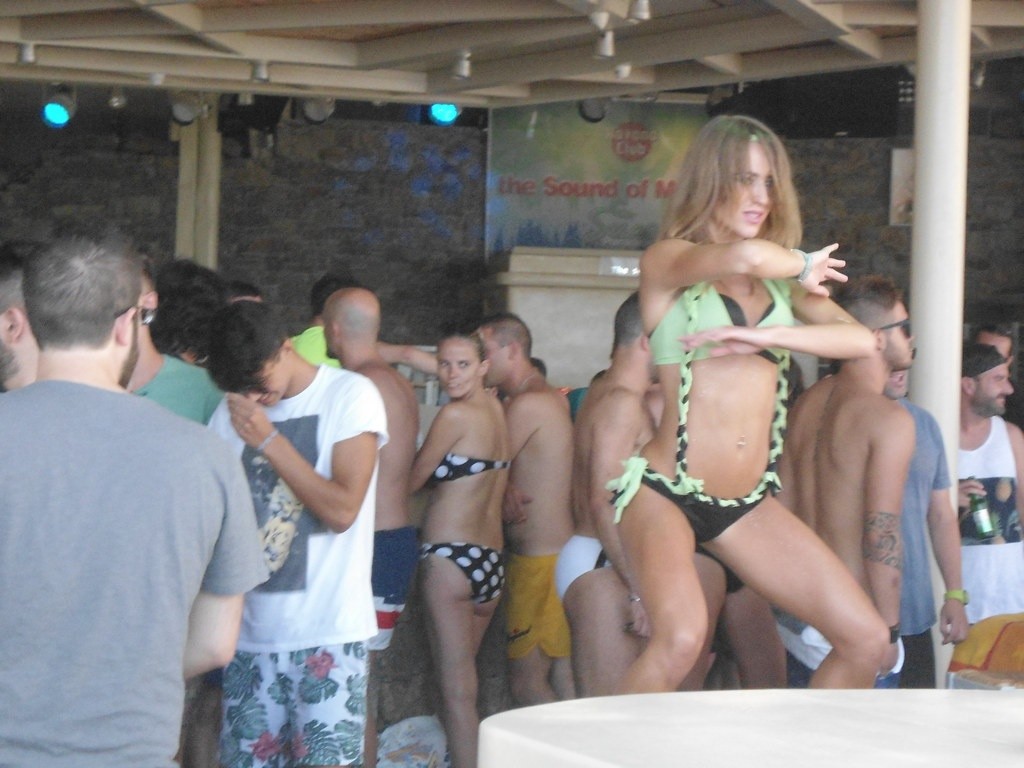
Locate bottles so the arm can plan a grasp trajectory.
[967,476,996,541]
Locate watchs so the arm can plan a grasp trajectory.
[942,590,969,605]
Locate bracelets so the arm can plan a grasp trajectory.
[890,622,901,642]
[628,598,641,602]
[791,247,812,282]
[257,429,278,453]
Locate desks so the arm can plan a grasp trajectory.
[477,688,1024,768]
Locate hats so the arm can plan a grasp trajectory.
[963,343,1007,378]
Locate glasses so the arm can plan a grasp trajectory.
[115,302,156,326]
[877,320,912,339]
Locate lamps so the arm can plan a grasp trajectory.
[19,0,651,128]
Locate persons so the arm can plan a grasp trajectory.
[1,211,666,768]
[767,273,1024,690]
[614,100,896,688]
